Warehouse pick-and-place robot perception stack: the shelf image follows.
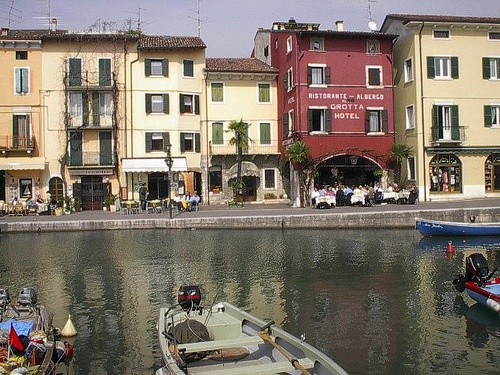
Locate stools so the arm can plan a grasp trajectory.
[122,208,139,215]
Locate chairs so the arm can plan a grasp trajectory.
[336,190,353,206]
[146,201,154,214]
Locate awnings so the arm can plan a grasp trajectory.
[121,157,187,172]
[226,161,261,179]
[0,156,45,170]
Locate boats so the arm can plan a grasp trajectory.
[465,251,500,313]
[414,215,500,238]
[156,285,349,375]
[0,285,55,373]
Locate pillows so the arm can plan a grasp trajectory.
[209,346,250,361]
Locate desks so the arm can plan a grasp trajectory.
[350,194,367,207]
[150,200,162,214]
[121,201,140,215]
[383,192,410,205]
[314,196,336,209]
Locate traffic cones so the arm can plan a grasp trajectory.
[446,243,454,252]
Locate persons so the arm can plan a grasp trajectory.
[139,183,147,210]
[13,195,56,214]
[177,190,200,213]
[311,183,419,207]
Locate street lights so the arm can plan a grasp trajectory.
[164,142,175,218]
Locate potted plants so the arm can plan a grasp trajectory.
[101,193,120,213]
[46,190,82,217]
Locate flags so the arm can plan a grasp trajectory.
[9,324,23,350]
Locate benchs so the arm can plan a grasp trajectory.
[185,354,265,375]
